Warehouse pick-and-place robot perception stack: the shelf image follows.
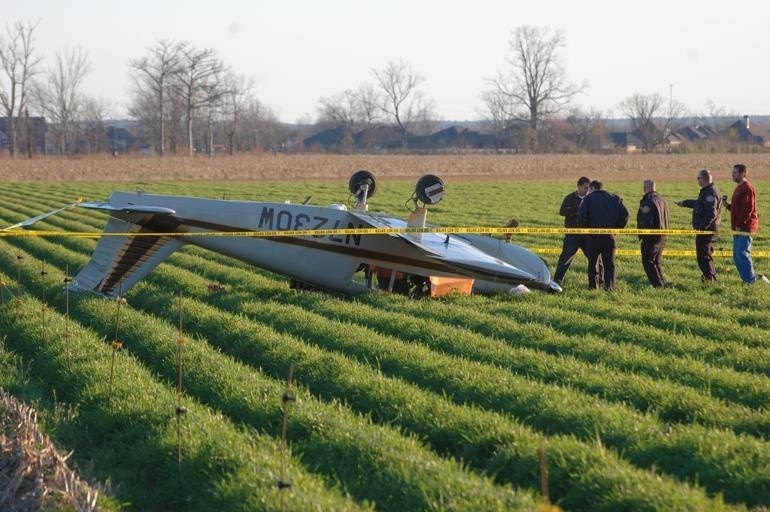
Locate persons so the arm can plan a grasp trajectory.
[722,164,759,287]
[552,176,606,290]
[637,179,669,290]
[676,168,722,284]
[577,179,629,293]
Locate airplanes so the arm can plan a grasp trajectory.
[64,189,563,305]
